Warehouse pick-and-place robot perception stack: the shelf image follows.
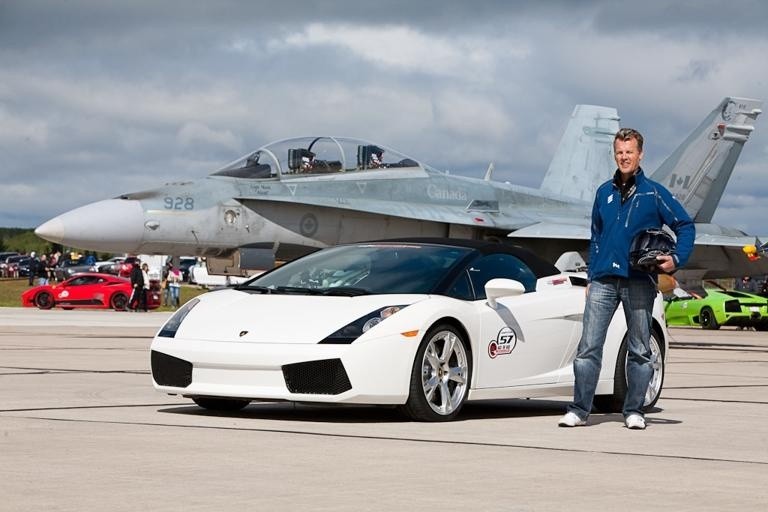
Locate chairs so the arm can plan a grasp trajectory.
[473,256,532,299]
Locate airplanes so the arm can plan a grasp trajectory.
[33,95,768,278]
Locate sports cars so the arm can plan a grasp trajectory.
[148,238,668,421]
[663,284,768,331]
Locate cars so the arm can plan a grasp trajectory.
[0,252,275,311]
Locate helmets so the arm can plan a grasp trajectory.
[628,228,676,265]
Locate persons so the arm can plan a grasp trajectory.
[0,260,20,279]
[734,272,760,295]
[557,127,697,428]
[27,246,183,311]
[760,277,768,297]
[369,152,384,167]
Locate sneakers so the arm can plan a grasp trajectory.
[625,414,645,428]
[559,411,587,427]
